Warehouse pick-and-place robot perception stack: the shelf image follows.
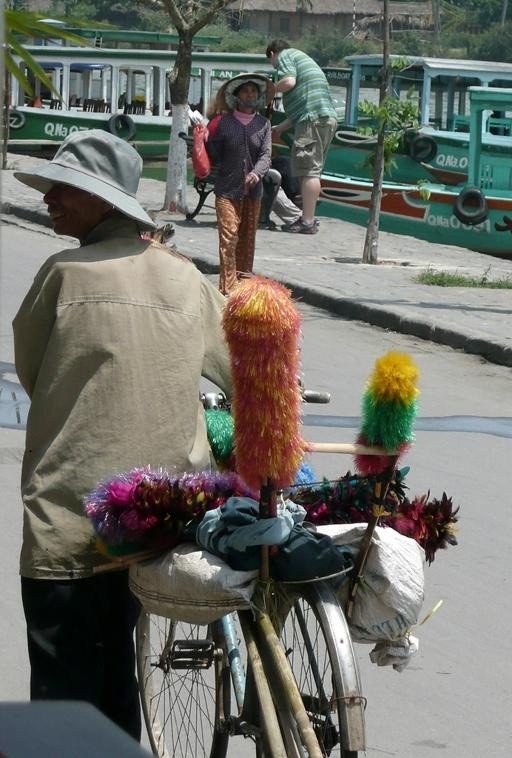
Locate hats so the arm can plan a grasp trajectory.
[216,73,276,116]
[13,129,158,230]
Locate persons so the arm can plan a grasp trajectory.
[260,151,320,233]
[266,39,336,234]
[11,128,237,745]
[193,73,277,298]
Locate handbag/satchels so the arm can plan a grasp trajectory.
[193,133,210,179]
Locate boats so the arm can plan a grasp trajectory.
[0,19,512,263]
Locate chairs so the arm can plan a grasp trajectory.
[50,92,148,116]
[178,130,280,231]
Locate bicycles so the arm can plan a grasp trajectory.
[133,386,370,757]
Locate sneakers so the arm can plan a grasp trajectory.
[281,213,320,234]
